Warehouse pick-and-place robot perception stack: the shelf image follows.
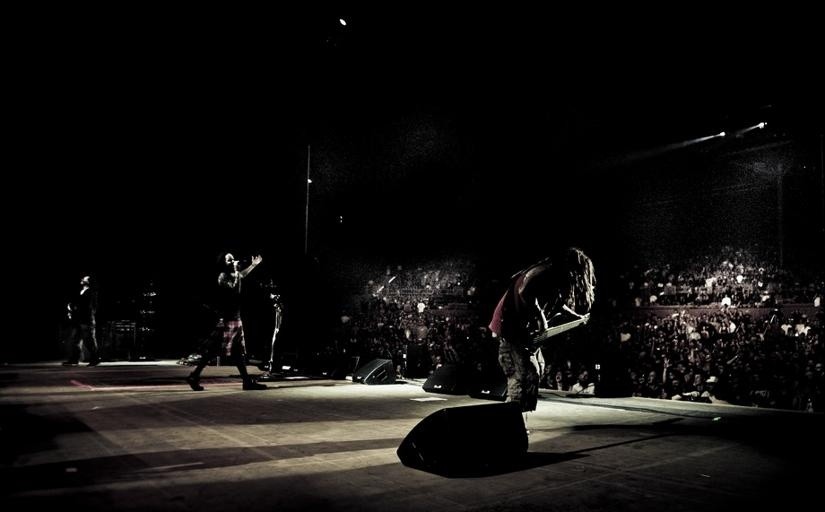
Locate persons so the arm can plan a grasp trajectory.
[183,252,269,389]
[62,274,103,368]
[306,106,825,415]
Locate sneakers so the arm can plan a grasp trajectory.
[187,372,202,389]
[243,380,267,389]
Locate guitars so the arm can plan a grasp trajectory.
[532,312,592,350]
